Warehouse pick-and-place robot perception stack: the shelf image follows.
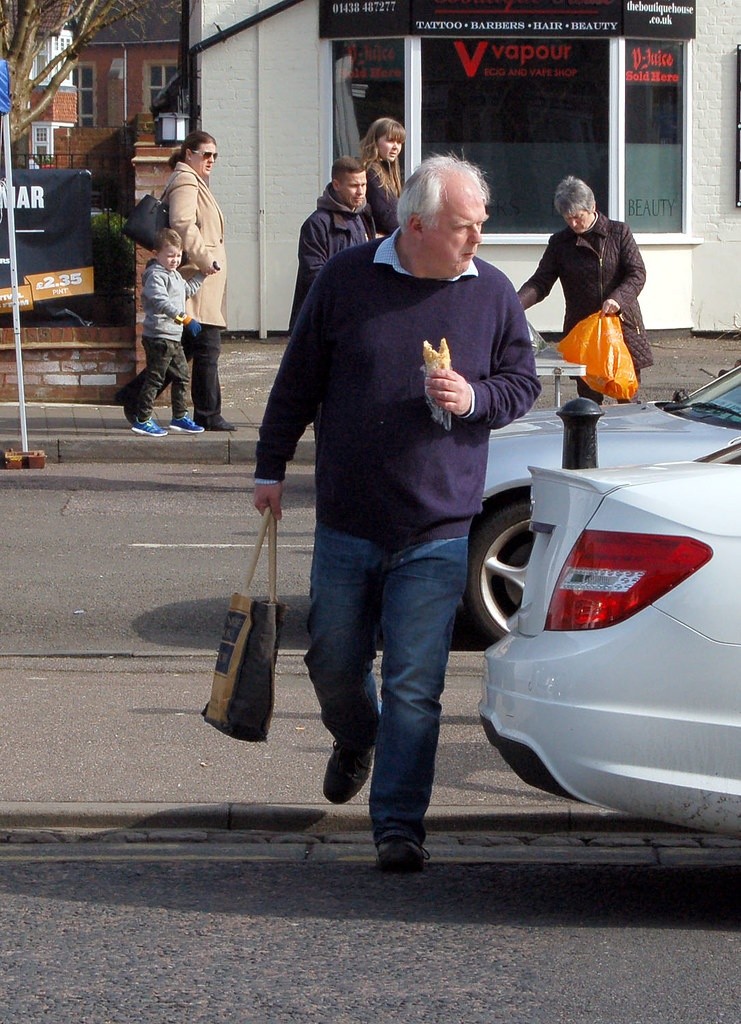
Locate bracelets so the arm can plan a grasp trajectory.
[174,311,187,325]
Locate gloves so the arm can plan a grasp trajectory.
[175,312,201,337]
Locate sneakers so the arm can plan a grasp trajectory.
[168,413,204,432]
[132,417,168,438]
[323,737,374,806]
[374,836,425,872]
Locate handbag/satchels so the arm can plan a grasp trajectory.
[202,506,288,742]
[556,309,638,402]
[123,195,172,252]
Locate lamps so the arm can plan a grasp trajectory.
[154,112,191,145]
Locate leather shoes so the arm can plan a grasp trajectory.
[116,387,136,424]
[195,417,236,432]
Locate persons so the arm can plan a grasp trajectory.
[517,178,654,405]
[114,131,236,436]
[289,117,407,334]
[252,156,541,872]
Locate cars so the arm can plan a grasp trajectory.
[479,436,741,845]
[462,357,741,644]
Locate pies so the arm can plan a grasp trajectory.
[422,337,450,370]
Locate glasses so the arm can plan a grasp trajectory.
[191,150,218,160]
